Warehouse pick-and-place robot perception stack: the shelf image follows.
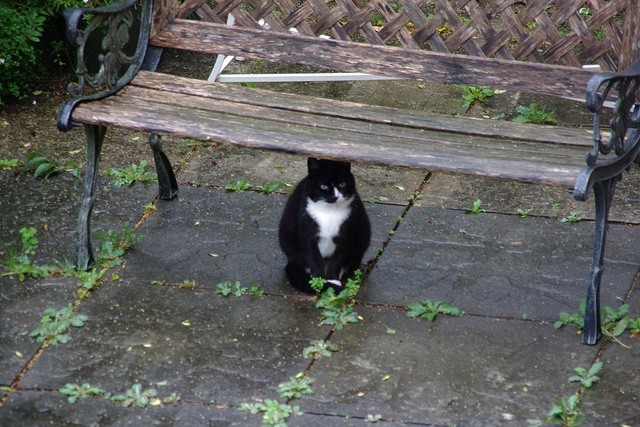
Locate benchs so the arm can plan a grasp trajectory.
[59,0,639,345]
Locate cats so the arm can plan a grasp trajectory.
[279,157,371,295]
